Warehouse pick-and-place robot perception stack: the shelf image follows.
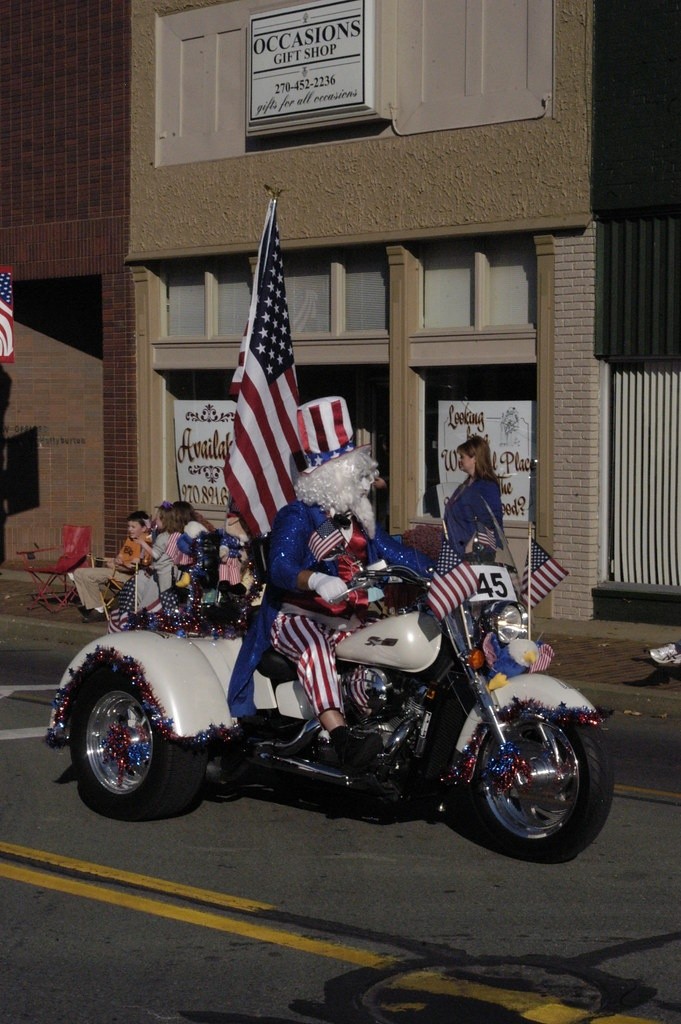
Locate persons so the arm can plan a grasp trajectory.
[72,510,153,623]
[132,500,215,594]
[442,435,505,565]
[262,397,442,771]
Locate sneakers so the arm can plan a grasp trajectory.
[650,644,681,670]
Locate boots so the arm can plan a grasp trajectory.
[330,723,384,772]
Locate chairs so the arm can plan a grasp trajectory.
[99,579,125,621]
[16,524,94,614]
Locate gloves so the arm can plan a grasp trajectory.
[306,570,353,606]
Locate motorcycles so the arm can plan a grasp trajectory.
[46,533,614,865]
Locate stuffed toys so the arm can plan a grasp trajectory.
[217,513,250,595]
[483,631,556,691]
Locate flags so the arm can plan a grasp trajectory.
[308,518,344,563]
[106,576,162,636]
[518,538,569,609]
[427,535,481,622]
[222,197,302,540]
[476,522,497,550]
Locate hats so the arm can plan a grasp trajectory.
[290,399,374,475]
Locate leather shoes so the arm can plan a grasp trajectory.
[83,608,107,623]
